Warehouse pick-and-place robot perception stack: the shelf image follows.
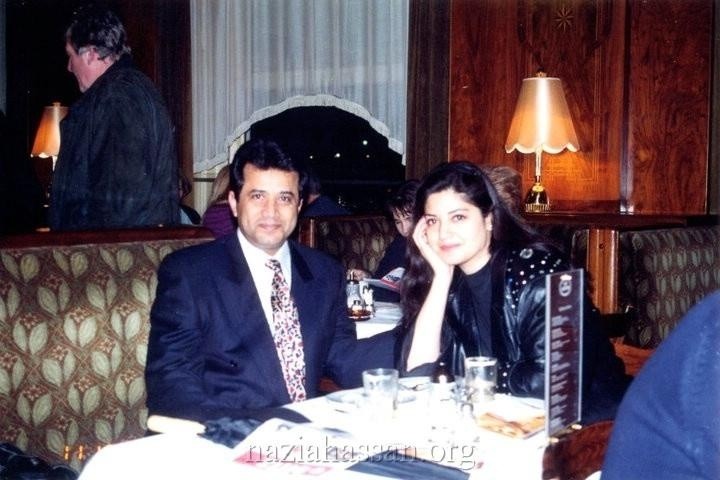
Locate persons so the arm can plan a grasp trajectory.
[175,169,202,226]
[49,7,182,231]
[143,140,366,437]
[296,162,347,216]
[202,165,238,240]
[483,165,526,226]
[599,287,720,480]
[391,161,629,430]
[347,177,428,305]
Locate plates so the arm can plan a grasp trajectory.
[325,389,416,405]
[474,393,547,441]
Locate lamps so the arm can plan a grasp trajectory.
[502,69,582,213]
[31,105,76,208]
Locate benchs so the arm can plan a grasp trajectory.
[2,211,720,480]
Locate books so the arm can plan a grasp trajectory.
[361,265,405,292]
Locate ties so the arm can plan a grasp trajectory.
[264,260,306,401]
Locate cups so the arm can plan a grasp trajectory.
[464,355,498,401]
[362,368,399,411]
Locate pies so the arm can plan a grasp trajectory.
[478,412,545,437]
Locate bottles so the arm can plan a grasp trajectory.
[347,271,376,315]
